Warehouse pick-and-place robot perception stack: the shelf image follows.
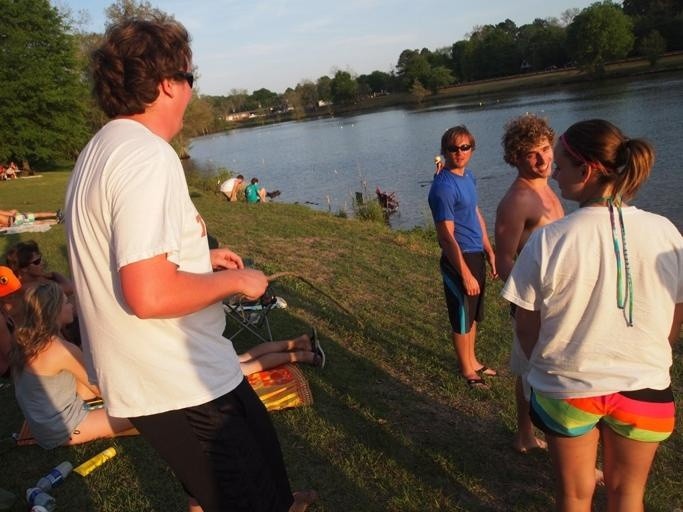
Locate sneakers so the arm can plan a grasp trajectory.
[56,208,64,224]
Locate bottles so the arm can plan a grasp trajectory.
[36,460,72,490]
[24,486,56,511]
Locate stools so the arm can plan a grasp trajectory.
[223,289,276,344]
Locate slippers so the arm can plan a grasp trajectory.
[311,326,325,368]
[477,366,497,376]
[468,377,491,390]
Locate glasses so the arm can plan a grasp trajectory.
[448,144,471,152]
[30,256,41,265]
[174,72,193,88]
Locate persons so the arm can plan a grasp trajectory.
[218,173,245,203]
[4,238,75,299]
[12,277,327,451]
[492,113,604,484]
[501,116,683,512]
[62,17,299,512]
[0,162,18,181]
[0,264,14,379]
[426,123,502,391]
[434,154,444,174]
[0,207,65,229]
[245,177,268,203]
